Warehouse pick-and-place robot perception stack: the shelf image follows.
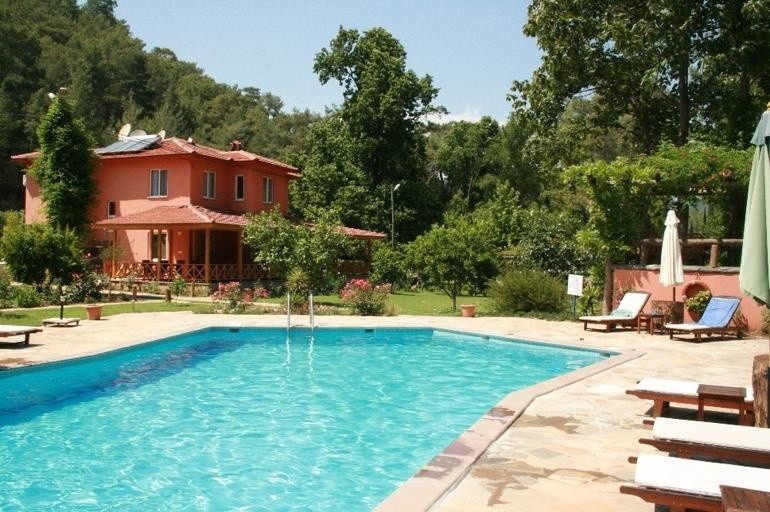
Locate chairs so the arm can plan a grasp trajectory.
[578,289,652,331]
[663,295,742,343]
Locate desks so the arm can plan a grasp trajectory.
[637,313,665,335]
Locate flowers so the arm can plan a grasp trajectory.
[339,277,392,316]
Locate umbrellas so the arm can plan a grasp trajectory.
[738,100,770,310]
[660,210,685,302]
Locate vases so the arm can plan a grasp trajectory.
[460,304,476,317]
[86,304,104,320]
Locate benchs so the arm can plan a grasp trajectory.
[620,376,770,512]
